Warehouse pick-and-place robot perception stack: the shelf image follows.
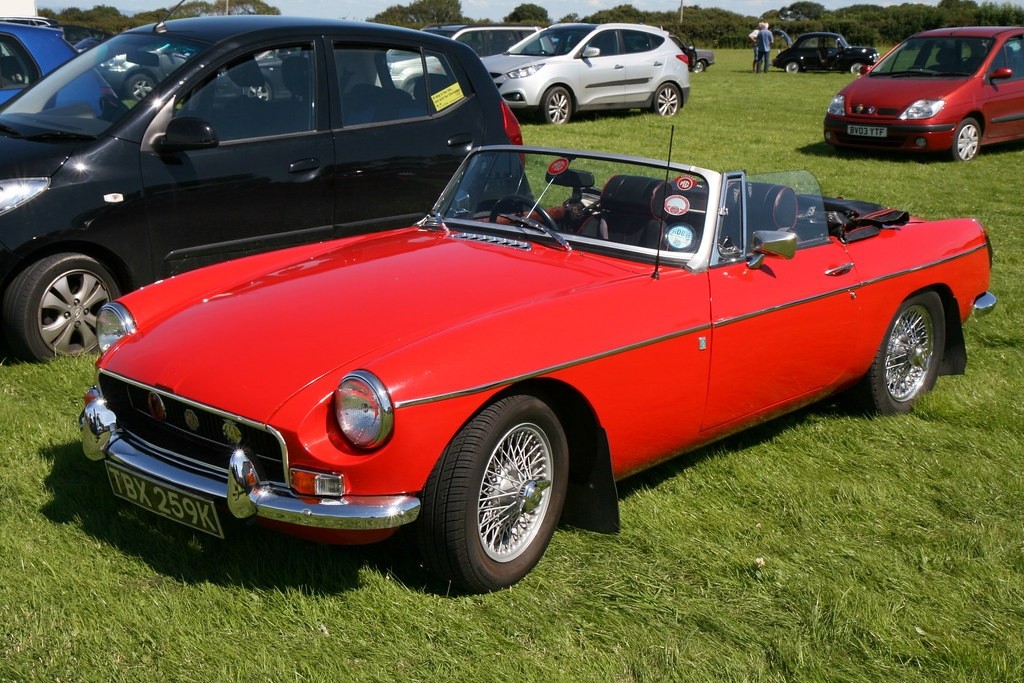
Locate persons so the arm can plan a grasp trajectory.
[748,22,765,70]
[758,22,773,73]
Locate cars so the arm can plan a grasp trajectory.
[769,26,880,75]
[822,25,1023,163]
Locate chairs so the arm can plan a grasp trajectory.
[574,174,675,251]
[926,45,965,73]
[209,55,260,136]
[967,43,1015,77]
[680,175,798,255]
[269,56,314,132]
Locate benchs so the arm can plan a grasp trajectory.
[342,76,446,127]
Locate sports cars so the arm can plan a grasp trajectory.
[76,142,998,594]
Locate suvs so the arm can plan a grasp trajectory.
[415,21,715,125]
[0,13,538,364]
